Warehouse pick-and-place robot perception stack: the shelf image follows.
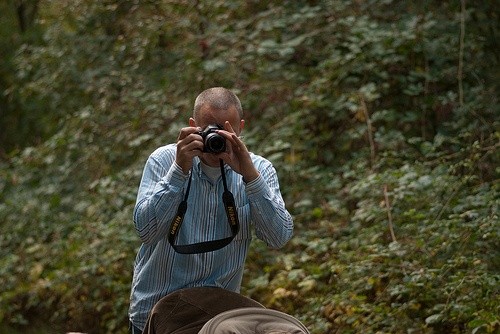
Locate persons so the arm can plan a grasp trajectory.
[128,87,294,334]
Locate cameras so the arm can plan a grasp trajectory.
[188,124,227,154]
